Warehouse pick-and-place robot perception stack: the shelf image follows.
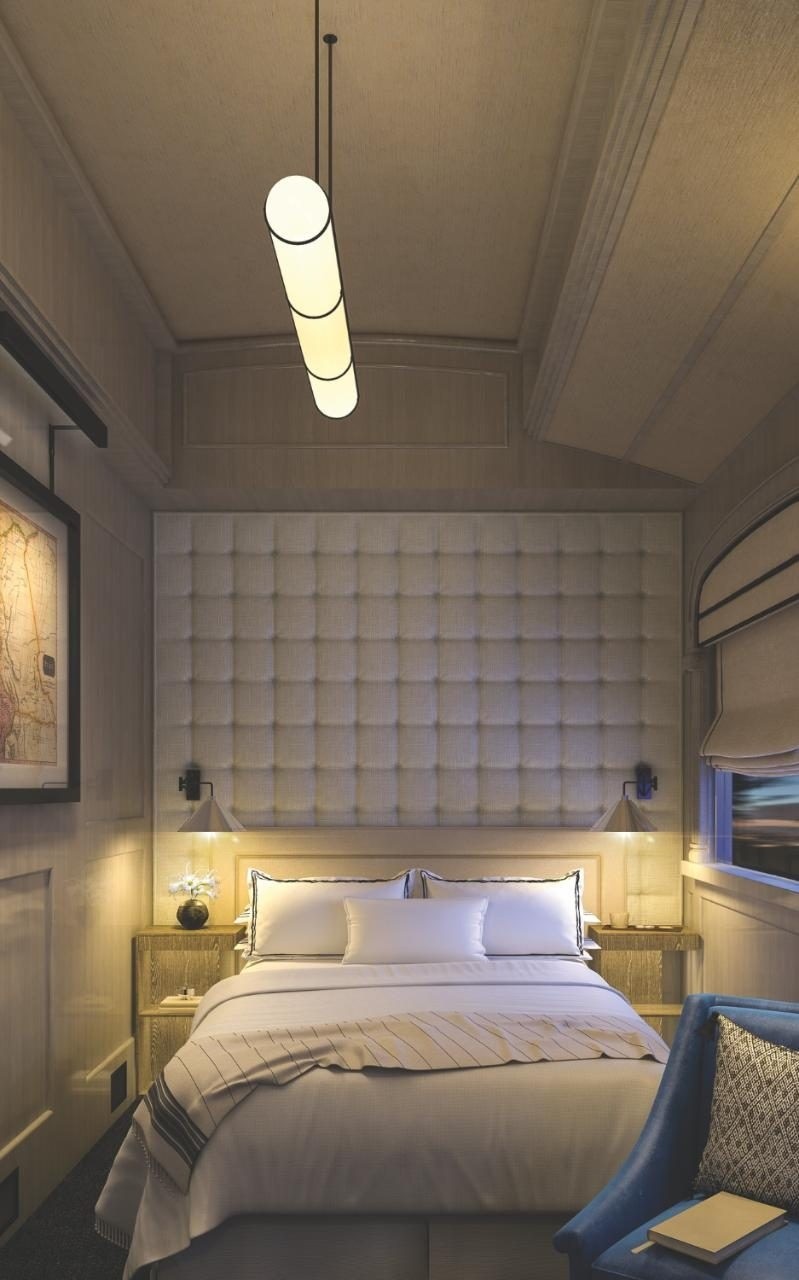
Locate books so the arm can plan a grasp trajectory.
[645,1190,789,1267]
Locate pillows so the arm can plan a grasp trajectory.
[416,868,594,961]
[690,1012,798,1217]
[341,895,489,966]
[234,867,417,961]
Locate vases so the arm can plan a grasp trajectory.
[177,899,208,929]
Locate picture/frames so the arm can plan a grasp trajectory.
[0,450,78,810]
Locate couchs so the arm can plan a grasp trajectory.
[553,990,799,1280]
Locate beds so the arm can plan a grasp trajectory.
[92,828,670,1280]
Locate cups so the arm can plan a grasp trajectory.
[608,911,629,930]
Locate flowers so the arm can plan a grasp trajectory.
[169,864,222,901]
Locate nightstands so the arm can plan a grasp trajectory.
[136,923,245,1099]
[588,924,700,1049]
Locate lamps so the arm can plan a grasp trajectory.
[179,770,246,833]
[264,0,361,418]
[590,765,661,834]
